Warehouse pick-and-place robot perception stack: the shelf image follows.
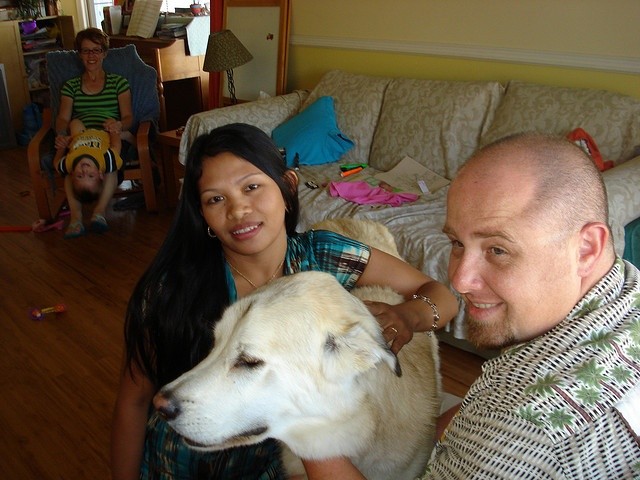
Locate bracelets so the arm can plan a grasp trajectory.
[410,294,440,337]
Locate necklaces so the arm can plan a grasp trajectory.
[224,257,285,289]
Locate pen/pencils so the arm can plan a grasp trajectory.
[294,153,300,171]
[340,166,363,177]
[340,164,367,170]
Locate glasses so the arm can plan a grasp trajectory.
[81,47,103,55]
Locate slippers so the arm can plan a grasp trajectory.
[90,214,108,232]
[63,222,85,239]
[33,218,64,233]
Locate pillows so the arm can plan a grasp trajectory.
[272,95,354,167]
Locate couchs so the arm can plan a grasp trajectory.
[29,45,160,224]
[177,70,639,362]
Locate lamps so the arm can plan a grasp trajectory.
[203,31,253,106]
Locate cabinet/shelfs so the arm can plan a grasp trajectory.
[16,15,75,118]
[100,17,209,131]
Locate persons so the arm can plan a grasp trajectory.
[107,122,459,480]
[53,119,124,222]
[52,27,133,238]
[300,132,639,479]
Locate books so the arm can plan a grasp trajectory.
[126,0,163,39]
[103,5,122,36]
[156,23,187,37]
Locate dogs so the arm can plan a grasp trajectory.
[152,217,441,480]
